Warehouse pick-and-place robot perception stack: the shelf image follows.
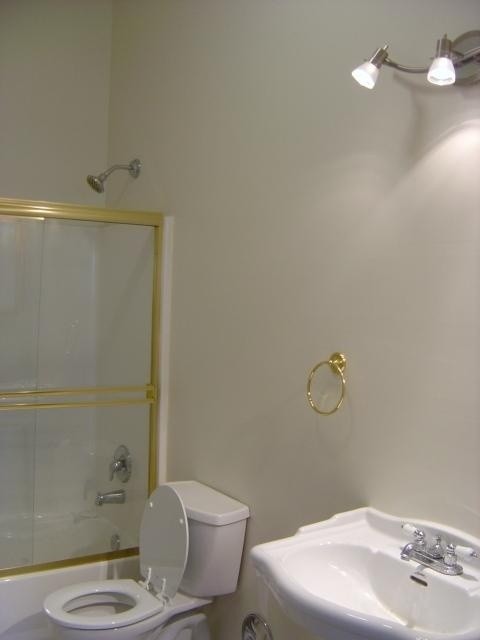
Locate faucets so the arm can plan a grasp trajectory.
[95,489,125,506]
[400,532,444,561]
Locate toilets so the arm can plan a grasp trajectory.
[41,480,249,640]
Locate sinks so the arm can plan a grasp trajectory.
[250,503,479,639]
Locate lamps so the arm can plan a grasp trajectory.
[351,28,479,91]
